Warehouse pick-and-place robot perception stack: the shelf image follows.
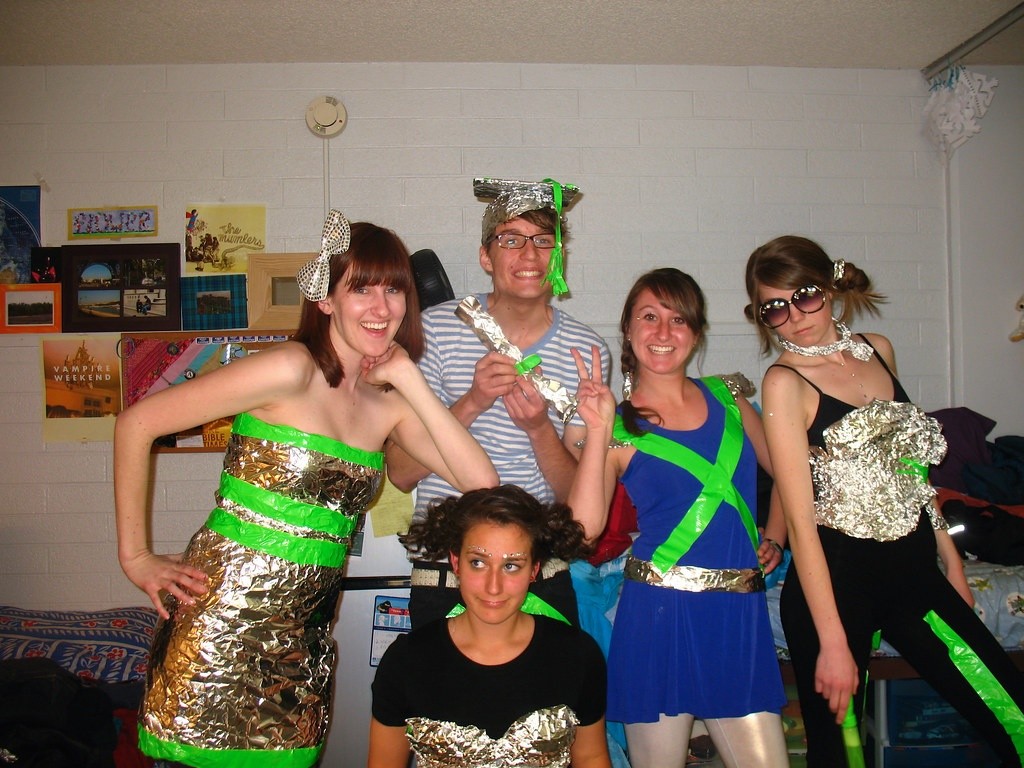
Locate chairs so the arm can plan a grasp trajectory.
[857,657,991,768]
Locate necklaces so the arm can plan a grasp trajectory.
[778,316,875,363]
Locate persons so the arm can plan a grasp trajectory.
[386,175,614,628]
[744,235,1024,768]
[114,209,501,768]
[568,267,789,768]
[136,296,151,315]
[367,484,612,768]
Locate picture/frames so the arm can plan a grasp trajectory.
[0,283,62,335]
[60,243,181,332]
[182,274,249,331]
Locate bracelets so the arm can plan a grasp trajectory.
[764,538,784,559]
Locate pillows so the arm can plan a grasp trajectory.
[868,558,1024,658]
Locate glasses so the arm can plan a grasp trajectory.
[484,232,556,249]
[757,282,828,329]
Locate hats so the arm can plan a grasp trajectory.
[473,177,578,295]
